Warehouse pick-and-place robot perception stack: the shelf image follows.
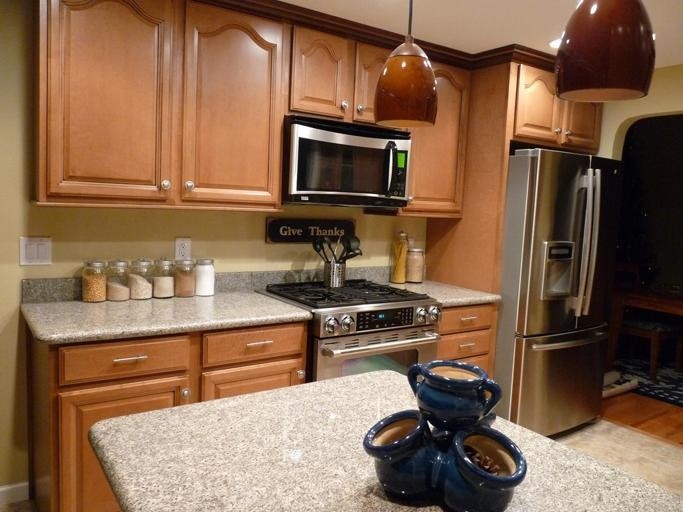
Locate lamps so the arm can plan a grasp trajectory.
[370,1,441,128]
[548,0,658,107]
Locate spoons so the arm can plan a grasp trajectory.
[324,236,339,264]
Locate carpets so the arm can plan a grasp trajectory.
[605,354,682,411]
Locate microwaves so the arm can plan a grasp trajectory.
[283,113,412,213]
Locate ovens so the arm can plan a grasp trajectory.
[307,325,440,383]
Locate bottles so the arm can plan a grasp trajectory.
[80,261,107,303]
[153,259,175,298]
[193,258,215,297]
[389,230,408,284]
[406,249,424,283]
[172,260,196,299]
[105,261,131,302]
[128,260,154,300]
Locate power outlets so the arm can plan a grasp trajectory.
[174,236,191,261]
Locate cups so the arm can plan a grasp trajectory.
[362,360,528,512]
[323,260,345,288]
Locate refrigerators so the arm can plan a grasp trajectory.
[493,146,622,439]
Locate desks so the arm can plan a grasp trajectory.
[598,285,682,381]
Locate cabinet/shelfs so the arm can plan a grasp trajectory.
[460,63,609,155]
[23,324,310,511]
[28,2,289,217]
[289,24,419,131]
[433,305,498,389]
[360,43,471,220]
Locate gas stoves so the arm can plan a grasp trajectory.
[257,278,442,339]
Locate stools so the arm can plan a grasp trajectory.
[616,314,672,380]
